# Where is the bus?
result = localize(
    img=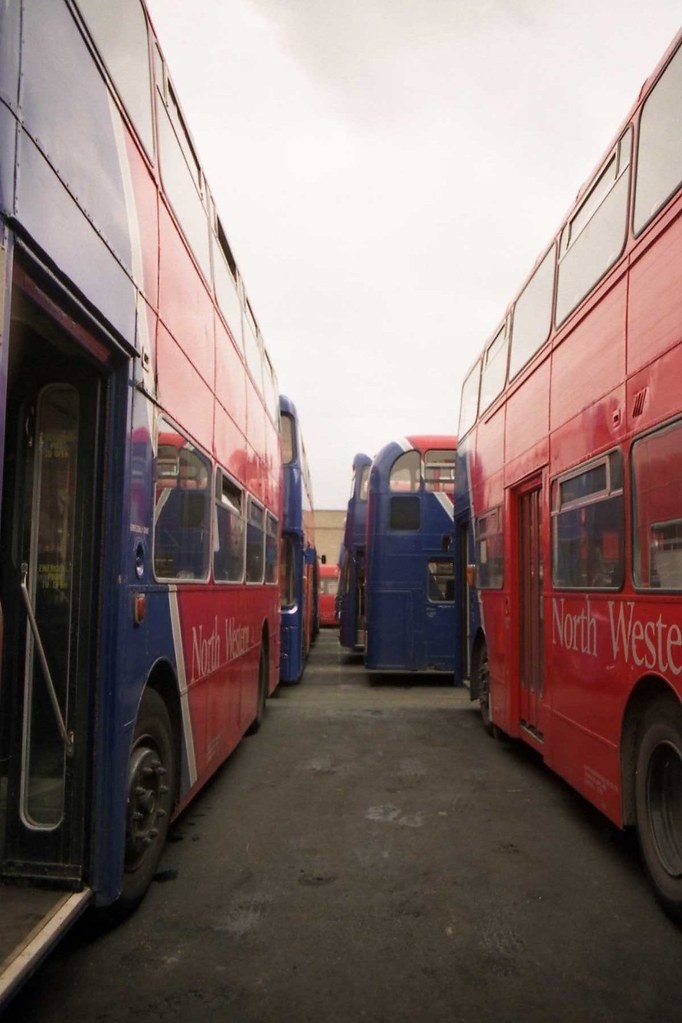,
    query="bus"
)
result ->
[335,431,459,680]
[559,451,662,596]
[455,25,682,907]
[278,398,327,684]
[131,427,275,594]
[316,563,342,629]
[1,2,278,998]
